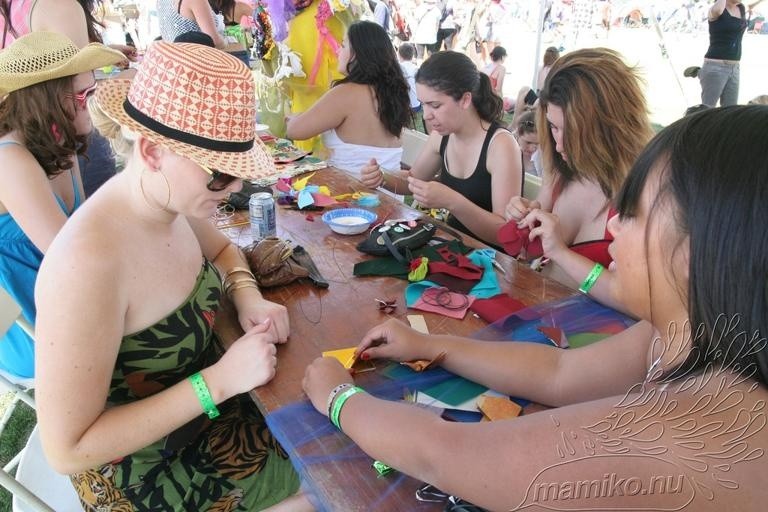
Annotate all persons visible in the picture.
[286,20,418,204]
[29,40,316,511]
[302,106,768,512]
[1,32,129,391]
[504,48,656,320]
[360,49,525,255]
[0,1,768,108]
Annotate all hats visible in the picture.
[96,41,276,180]
[0,28,127,96]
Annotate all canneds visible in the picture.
[248,191,276,242]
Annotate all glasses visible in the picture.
[199,166,237,192]
[64,82,98,107]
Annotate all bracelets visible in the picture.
[576,260,605,296]
[325,378,368,431]
[183,365,222,423]
[331,384,365,439]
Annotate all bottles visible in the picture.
[125,31,135,47]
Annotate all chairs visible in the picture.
[1,285,86,512]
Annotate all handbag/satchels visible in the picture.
[243,236,311,287]
[357,219,437,257]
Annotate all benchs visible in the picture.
[400,125,541,205]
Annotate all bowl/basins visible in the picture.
[322,208,378,235]
[255,123,269,137]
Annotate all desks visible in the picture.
[195,143,632,512]
